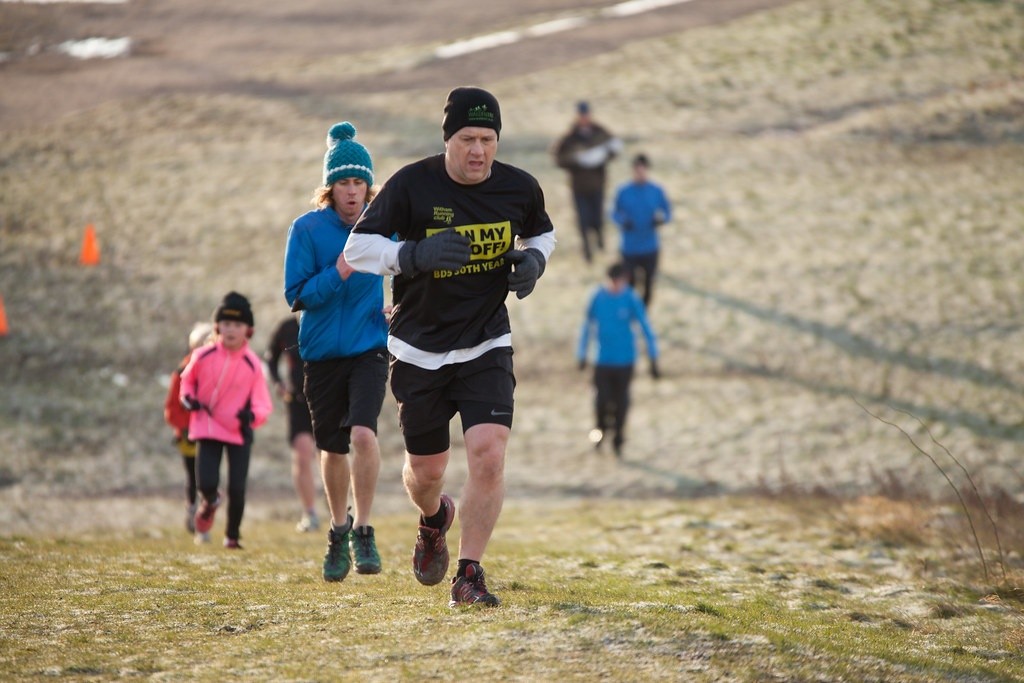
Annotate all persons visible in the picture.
[262,310,323,537]
[553,100,622,261]
[177,289,276,550]
[611,153,670,307]
[164,321,215,533]
[577,262,659,453]
[342,86,557,609]
[283,120,400,582]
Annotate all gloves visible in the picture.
[505,247,546,299]
[399,228,472,280]
[182,394,200,410]
[238,410,254,423]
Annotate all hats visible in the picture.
[321,122,373,188]
[216,292,254,326]
[442,87,502,141]
[189,323,214,350]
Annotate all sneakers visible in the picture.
[185,501,198,533]
[413,491,455,585]
[322,514,354,583]
[449,561,498,608]
[347,526,383,575]
[223,535,242,550]
[296,513,319,533]
[194,491,222,533]
[194,529,213,546]
